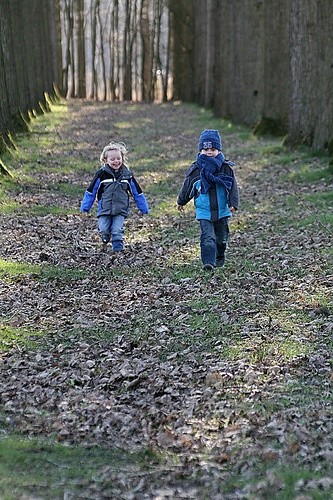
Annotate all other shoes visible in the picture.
[214,253,224,267]
[202,264,214,270]
[102,233,110,243]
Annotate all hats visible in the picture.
[198,129,222,152]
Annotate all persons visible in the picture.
[78,141,148,257]
[176,129,239,271]
